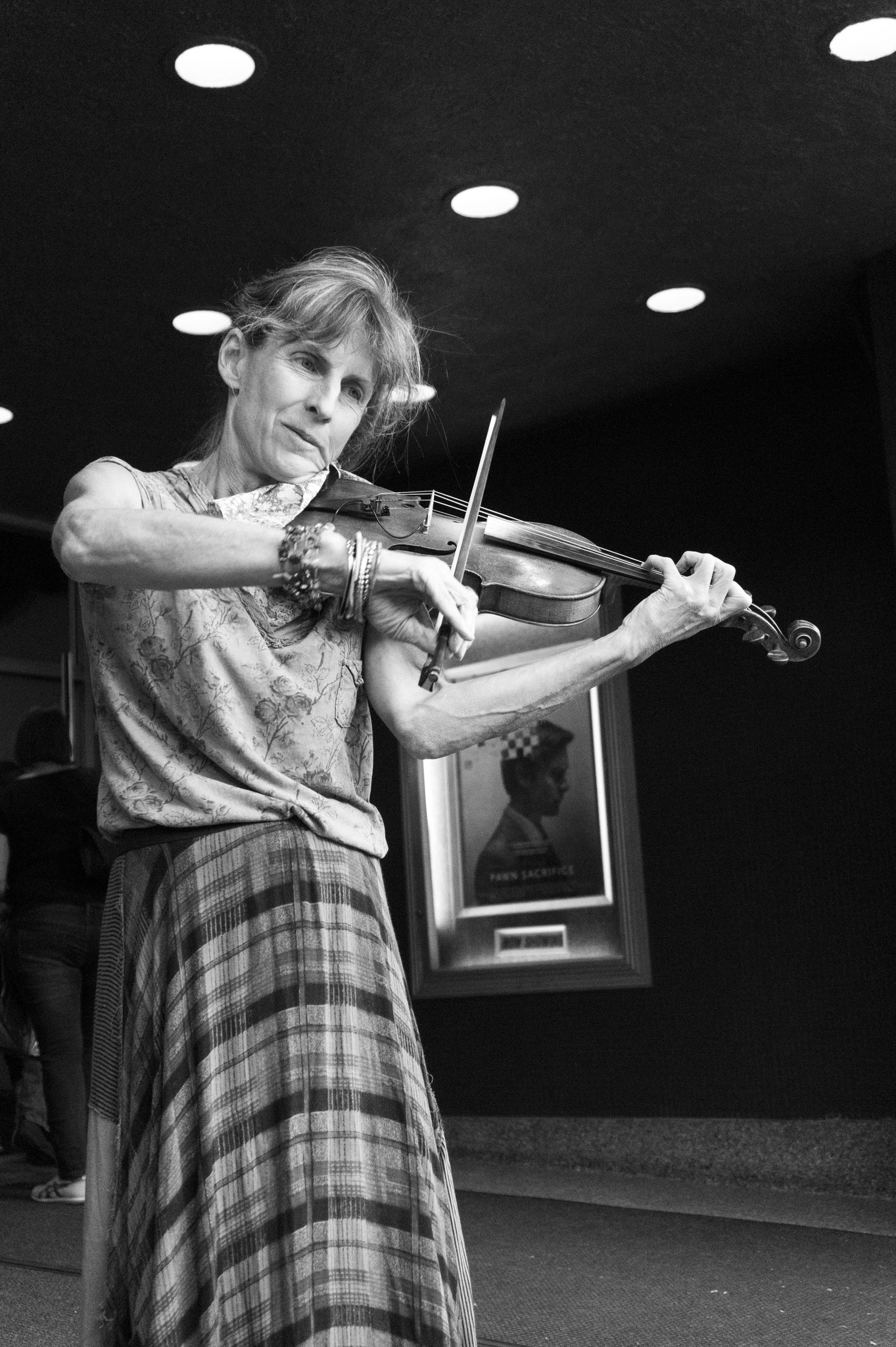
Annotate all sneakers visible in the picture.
[31,1174,87,1205]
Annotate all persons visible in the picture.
[0,713,95,1205]
[50,243,755,1347]
[474,720,580,906]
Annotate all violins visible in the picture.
[280,476,822,664]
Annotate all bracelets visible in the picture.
[282,522,382,625]
